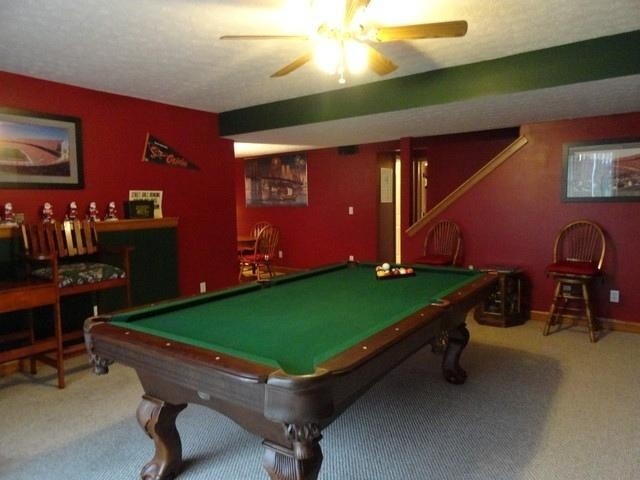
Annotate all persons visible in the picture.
[69,200,79,220]
[42,202,54,223]
[105,202,118,218]
[86,202,99,222]
[3,202,15,224]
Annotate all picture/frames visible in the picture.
[0,105,84,189]
[561,138,640,202]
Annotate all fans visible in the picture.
[219,1,467,77]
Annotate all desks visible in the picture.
[475,264,523,325]
[81,259,502,480]
[234,236,256,275]
[0,276,64,389]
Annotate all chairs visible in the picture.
[239,224,281,282]
[22,220,132,377]
[250,222,269,242]
[416,219,461,266]
[542,221,605,342]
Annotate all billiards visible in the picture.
[376,263,413,276]
